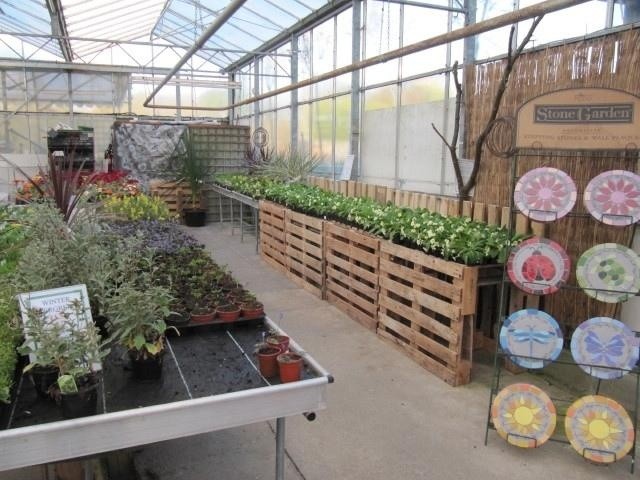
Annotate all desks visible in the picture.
[1,314,335,479]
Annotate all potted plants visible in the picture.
[103,291,175,379]
[56,301,121,418]
[209,170,304,209]
[256,334,305,383]
[3,124,269,337]
[18,308,71,398]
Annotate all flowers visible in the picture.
[307,184,533,267]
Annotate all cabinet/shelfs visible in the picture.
[484,87,640,476]
